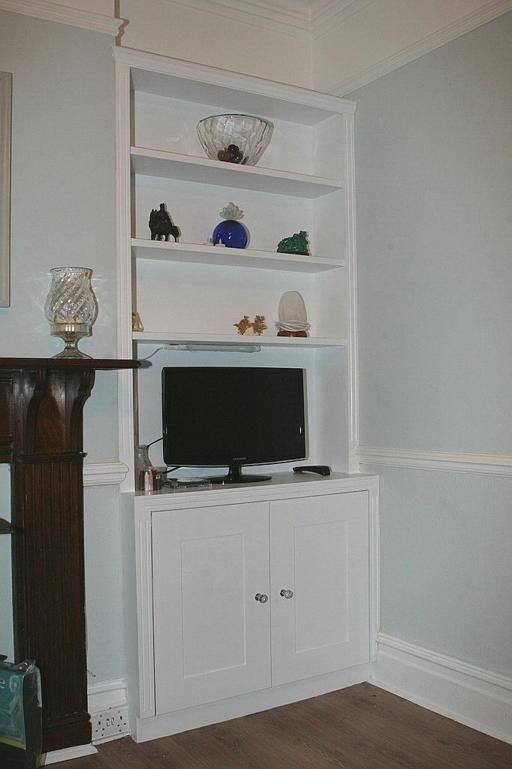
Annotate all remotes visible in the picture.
[293,466,330,476]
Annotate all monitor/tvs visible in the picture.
[161,365,309,484]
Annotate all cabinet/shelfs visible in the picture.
[112,42,364,492]
[127,471,379,743]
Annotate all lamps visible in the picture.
[45,265,97,360]
